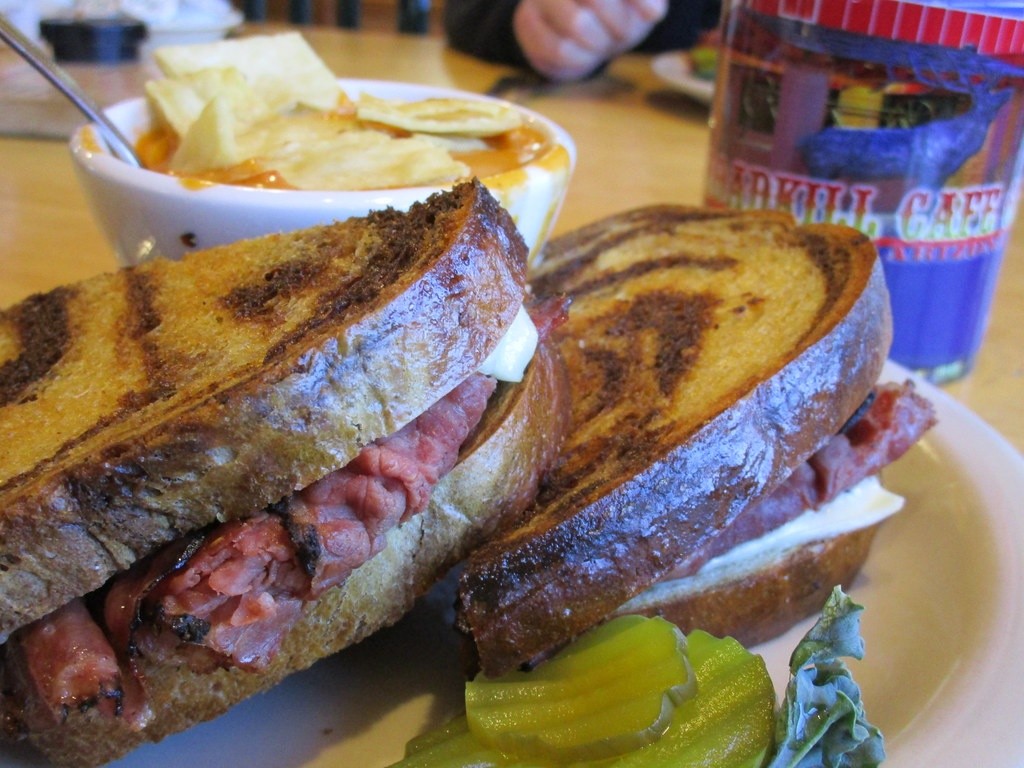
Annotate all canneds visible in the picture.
[696,0,1024,386]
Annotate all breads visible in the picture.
[0,181,896,768]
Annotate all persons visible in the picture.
[439,0,723,84]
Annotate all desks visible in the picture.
[0,27,1023,454]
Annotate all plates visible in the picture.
[652,53,717,100]
[77,356,1024,768]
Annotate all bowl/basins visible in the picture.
[71,80,573,275]
[10,10,246,48]
